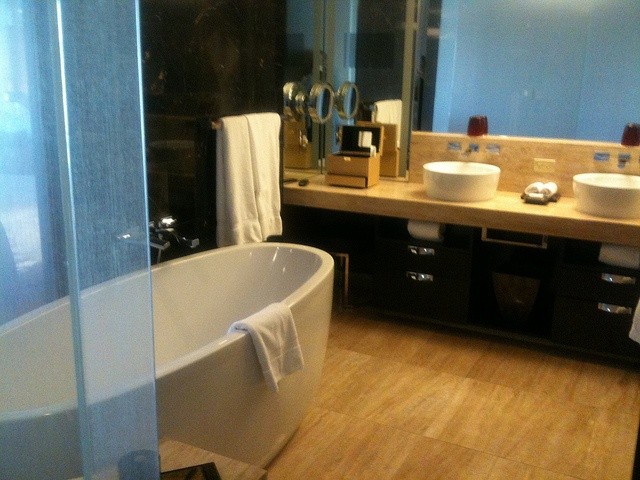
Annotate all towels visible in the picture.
[359,132,370,148]
[246,111,283,238]
[215,115,261,248]
[228,302,304,390]
[372,100,403,147]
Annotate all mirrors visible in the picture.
[320,0,417,179]
[337,123,383,156]
[410,1,640,144]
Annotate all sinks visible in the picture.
[572,173,640,218]
[423,160,501,200]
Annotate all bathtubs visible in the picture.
[0,244,334,480]
